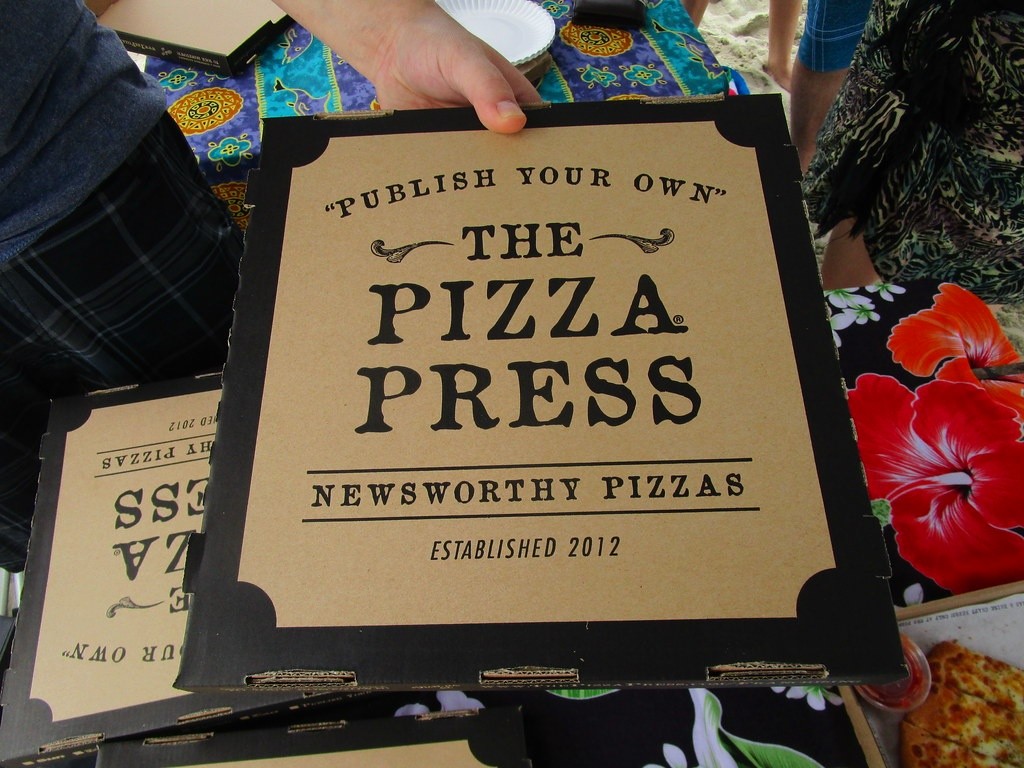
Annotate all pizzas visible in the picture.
[898,640,1024,768]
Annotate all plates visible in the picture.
[436,1,556,66]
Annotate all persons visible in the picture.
[684,0,1024,291]
[0,0,550,578]
